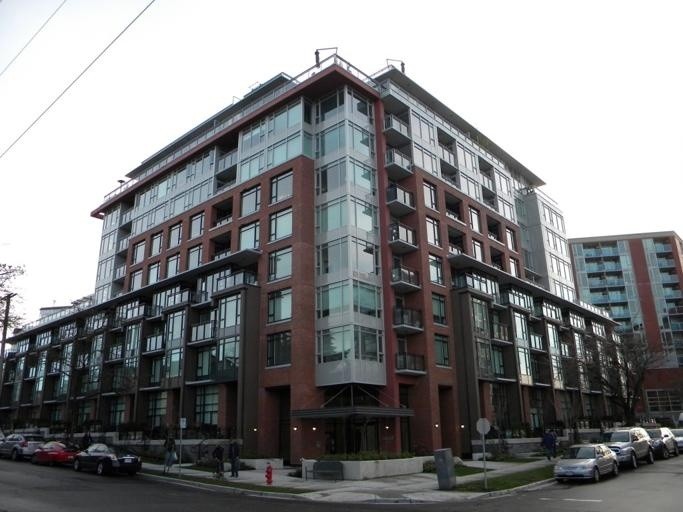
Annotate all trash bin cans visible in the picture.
[434,448,456,490]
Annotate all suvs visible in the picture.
[598,426,654,469]
[645,427,683,460]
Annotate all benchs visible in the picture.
[313,461,343,480]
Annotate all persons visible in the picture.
[227,441,240,479]
[212,442,225,479]
[540,428,555,460]
[161,432,178,476]
[79,433,90,450]
[550,429,560,455]
[323,429,336,454]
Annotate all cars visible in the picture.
[0,430,82,465]
[553,443,619,484]
[73,443,142,475]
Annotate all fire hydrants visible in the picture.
[265,465,273,486]
